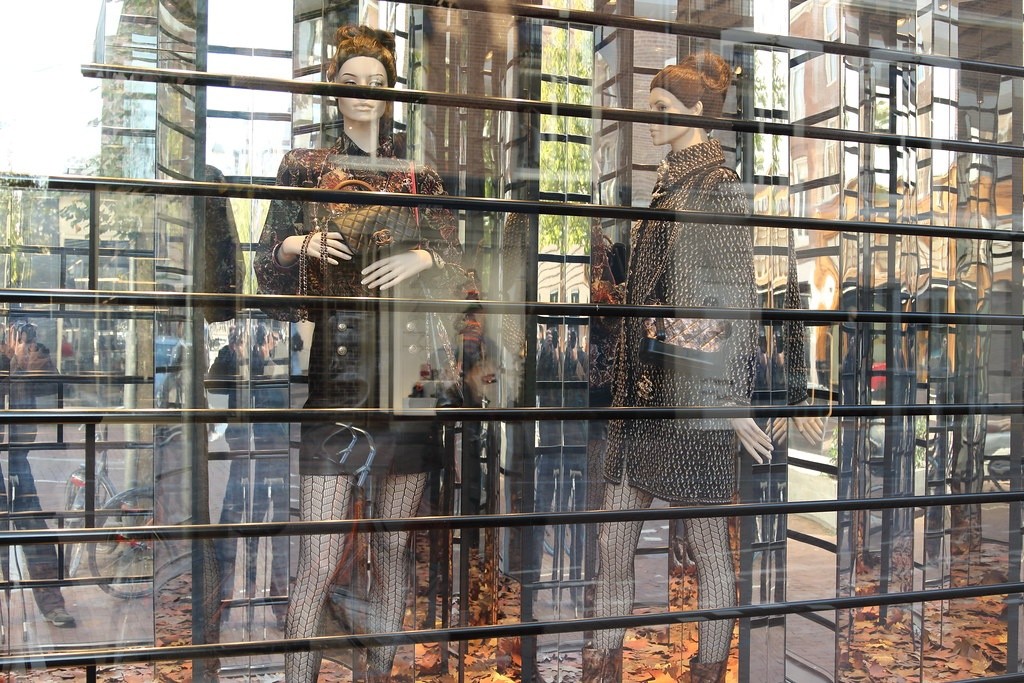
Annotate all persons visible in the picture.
[0,313,290,628]
[256,28,463,683]
[536,319,593,604]
[577,54,773,680]
[756,226,824,446]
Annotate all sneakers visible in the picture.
[42,607,75,626]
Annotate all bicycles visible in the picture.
[86,418,377,600]
[58,404,125,580]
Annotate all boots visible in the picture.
[689,657,728,683]
[582,641,623,683]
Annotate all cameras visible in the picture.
[232,324,265,345]
[11,322,35,343]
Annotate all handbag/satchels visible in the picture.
[328,180,424,258]
[638,297,732,376]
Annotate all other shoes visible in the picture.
[276,614,286,629]
